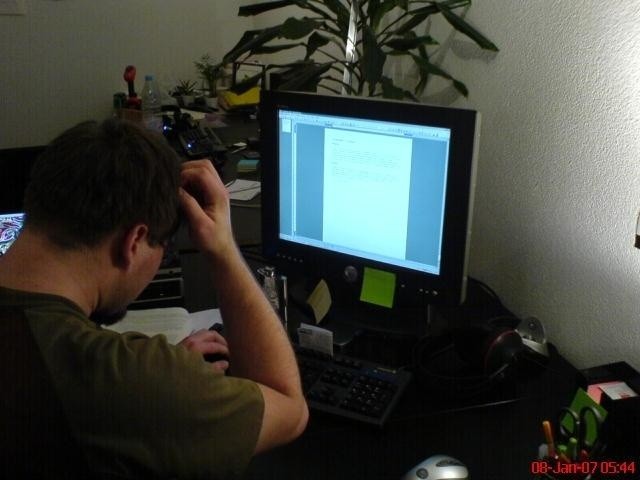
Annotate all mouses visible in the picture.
[400,454,471,480]
[178,118,198,129]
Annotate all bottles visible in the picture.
[141,75,163,136]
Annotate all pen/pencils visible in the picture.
[539,421,587,462]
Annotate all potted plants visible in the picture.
[170,77,198,107]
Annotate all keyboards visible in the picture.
[178,118,228,158]
[203,323,411,427]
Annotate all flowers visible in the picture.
[193,53,224,78]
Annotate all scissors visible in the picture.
[552,406,603,456]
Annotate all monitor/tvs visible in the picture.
[270,59,318,92]
[260,89,482,348]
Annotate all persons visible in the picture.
[0,119,312,479]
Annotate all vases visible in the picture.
[207,76,218,94]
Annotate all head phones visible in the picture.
[412,316,526,400]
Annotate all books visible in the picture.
[102,305,225,350]
